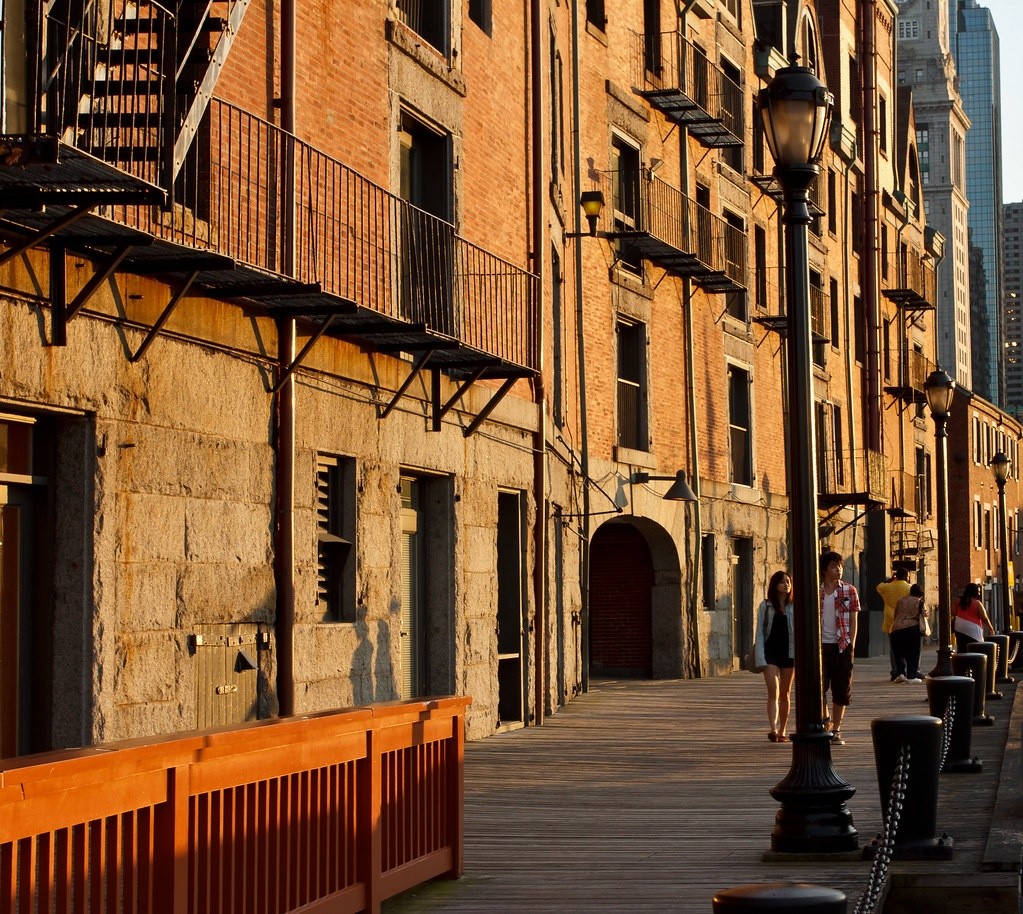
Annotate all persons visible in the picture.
[877,569,912,683]
[755,572,795,743]
[889,584,926,682]
[952,583,996,653]
[819,552,863,745]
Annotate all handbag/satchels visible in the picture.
[918,599,931,636]
[744,643,764,674]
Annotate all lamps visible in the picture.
[631,468,699,503]
[567,191,606,239]
[646,157,664,183]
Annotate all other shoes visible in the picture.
[778,736,787,742]
[822,716,831,731]
[917,673,925,679]
[768,732,777,742]
[829,736,845,744]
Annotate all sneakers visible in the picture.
[906,678,922,684]
[894,674,905,683]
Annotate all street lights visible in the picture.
[923,360,956,678]
[758,49,861,854]
[990,448,1013,633]
